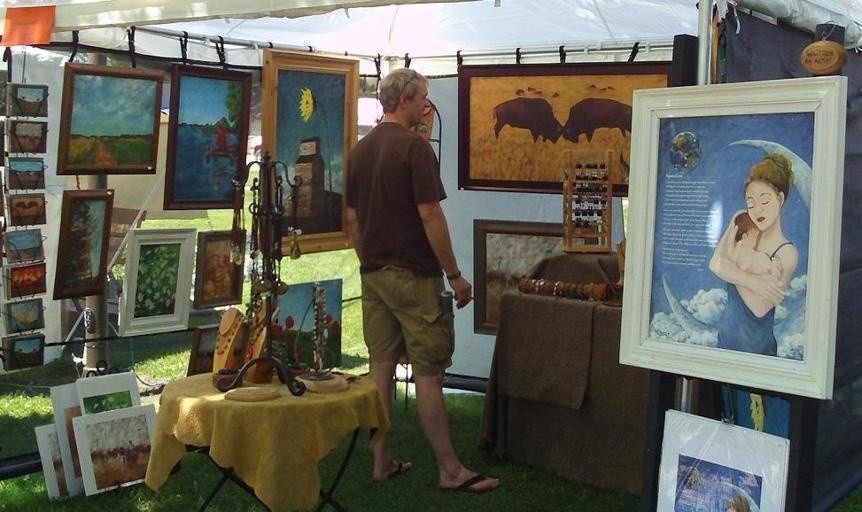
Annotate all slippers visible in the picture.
[440,473,499,493]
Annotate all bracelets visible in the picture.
[446,271,461,280]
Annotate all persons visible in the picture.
[341,70,505,489]
[708,151,799,355]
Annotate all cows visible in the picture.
[563,97,632,143]
[493,97,563,143]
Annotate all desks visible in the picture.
[144,369,392,512]
[477,290,650,498]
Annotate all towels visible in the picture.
[0,5,55,46]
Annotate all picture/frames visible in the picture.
[5,301,48,330]
[656,409,791,512]
[456,61,675,198]
[198,227,252,313]
[32,370,159,501]
[0,334,47,372]
[7,193,49,226]
[2,230,47,263]
[6,118,48,154]
[3,266,47,296]
[110,222,197,339]
[8,158,47,191]
[267,278,350,367]
[473,219,601,336]
[50,188,116,303]
[185,318,251,369]
[254,48,361,258]
[159,63,254,213]
[55,62,163,178]
[618,75,849,402]
[4,81,49,115]
